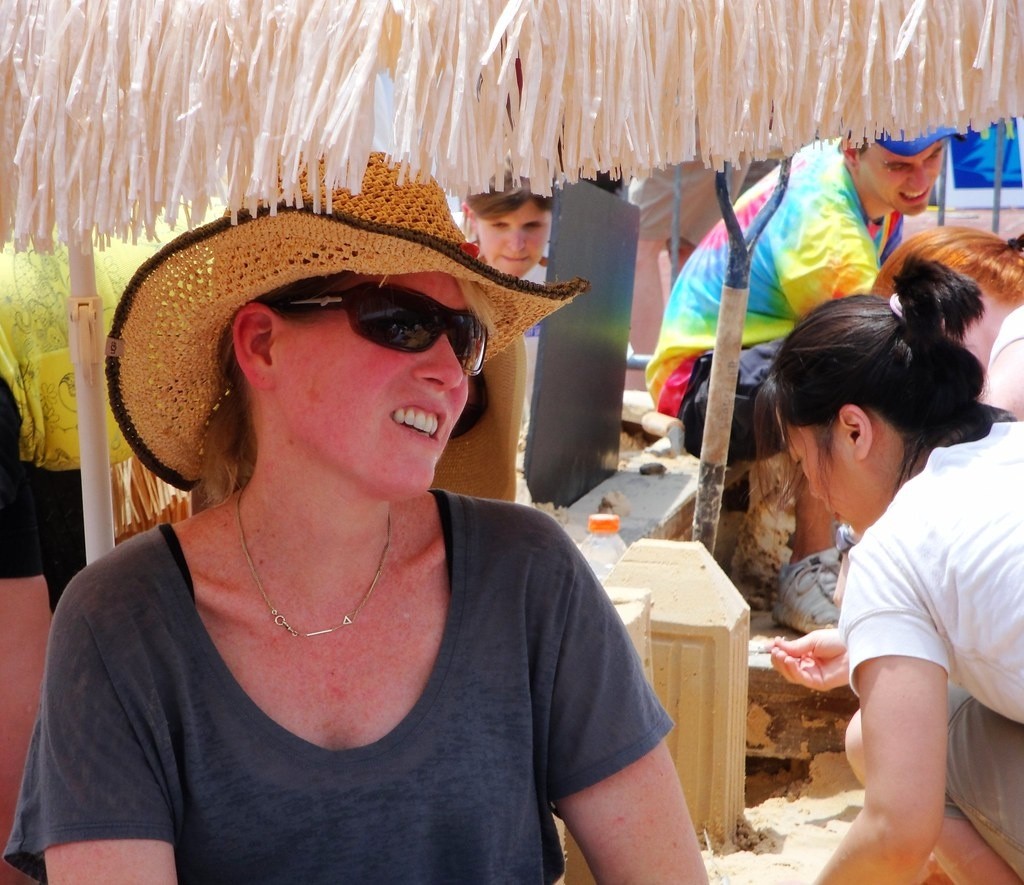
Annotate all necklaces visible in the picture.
[233,485,392,636]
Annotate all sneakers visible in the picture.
[772,546,844,634]
[836,524,856,553]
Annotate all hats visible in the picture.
[874,125,965,156]
[106,152,588,491]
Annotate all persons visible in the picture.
[0,375,55,883]
[462,172,552,338]
[643,125,1024,885]
[0,152,709,885]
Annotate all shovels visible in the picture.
[694,153,797,564]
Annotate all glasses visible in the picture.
[265,282,488,376]
